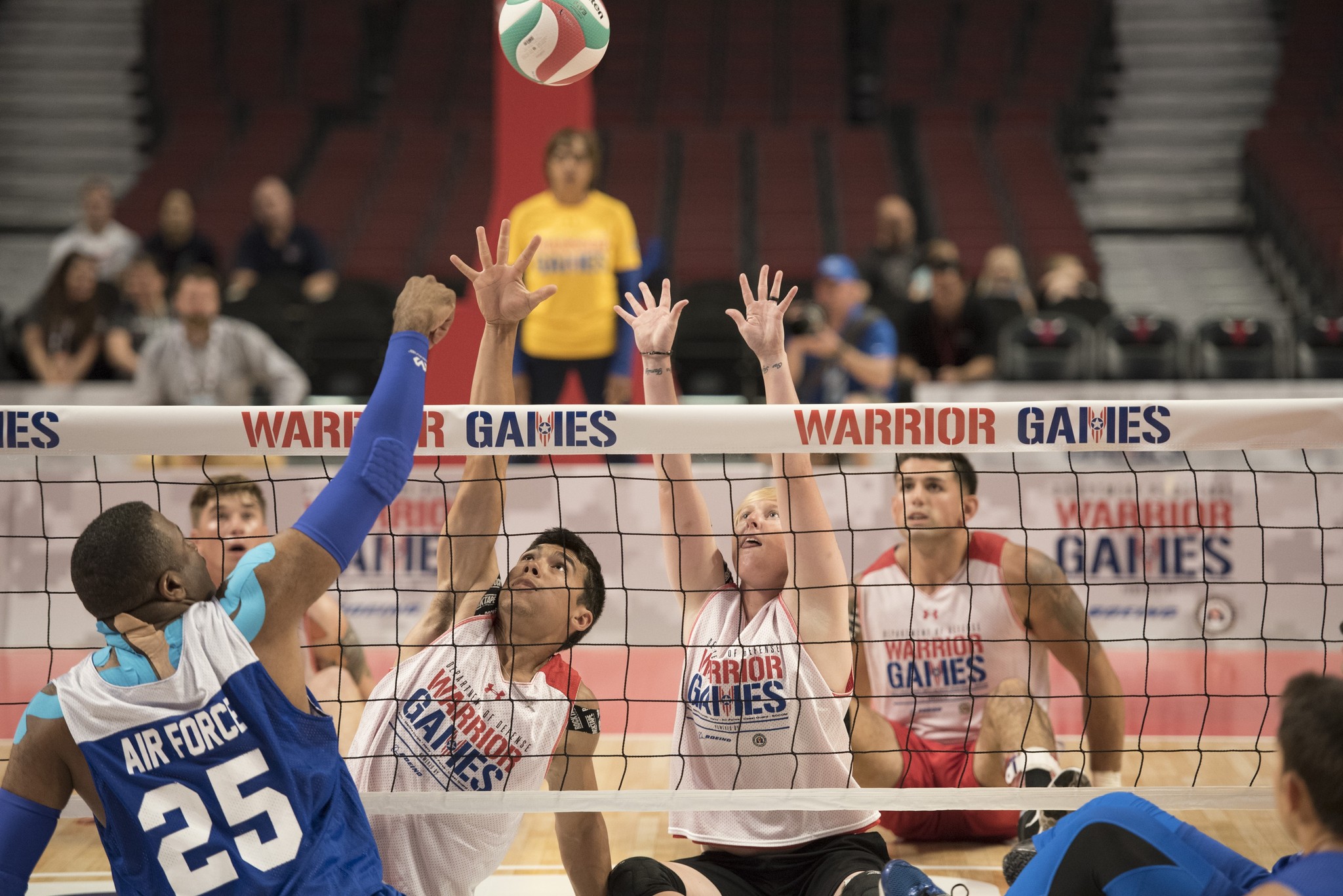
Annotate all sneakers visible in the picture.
[1003,839,1038,887]
[878,858,970,895]
[1017,768,1093,841]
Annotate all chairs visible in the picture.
[1242,0,1343,337]
[115,0,1102,314]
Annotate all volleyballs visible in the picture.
[498,0,610,87]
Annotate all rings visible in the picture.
[769,296,779,303]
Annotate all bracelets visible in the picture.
[640,349,674,357]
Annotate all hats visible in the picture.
[817,252,861,279]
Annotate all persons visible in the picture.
[2,175,339,407]
[784,193,1110,404]
[879,671,1343,896]
[605,265,889,896]
[844,453,1122,886]
[504,127,642,404]
[0,220,611,896]
